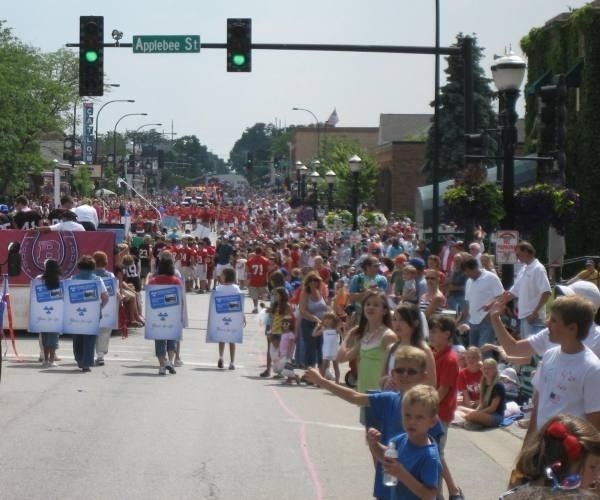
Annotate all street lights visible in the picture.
[71,81,123,169]
[131,123,164,187]
[113,113,149,169]
[292,107,321,158]
[292,159,337,222]
[348,153,362,230]
[93,99,136,164]
[150,131,179,170]
[489,42,529,305]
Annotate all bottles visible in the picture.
[383,443,398,484]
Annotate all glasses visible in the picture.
[393,367,421,375]
[425,276,436,280]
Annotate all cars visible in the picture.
[180,194,210,208]
[206,170,248,190]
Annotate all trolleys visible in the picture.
[344,353,357,387]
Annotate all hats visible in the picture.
[553,279,600,316]
[585,259,594,266]
[500,366,521,386]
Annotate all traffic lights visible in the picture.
[226,18,253,73]
[79,15,103,97]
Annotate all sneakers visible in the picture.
[217,358,224,368]
[157,357,185,376]
[38,352,61,368]
[127,282,281,330]
[76,356,105,371]
[228,363,235,370]
[259,368,343,387]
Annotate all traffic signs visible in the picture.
[132,35,201,55]
